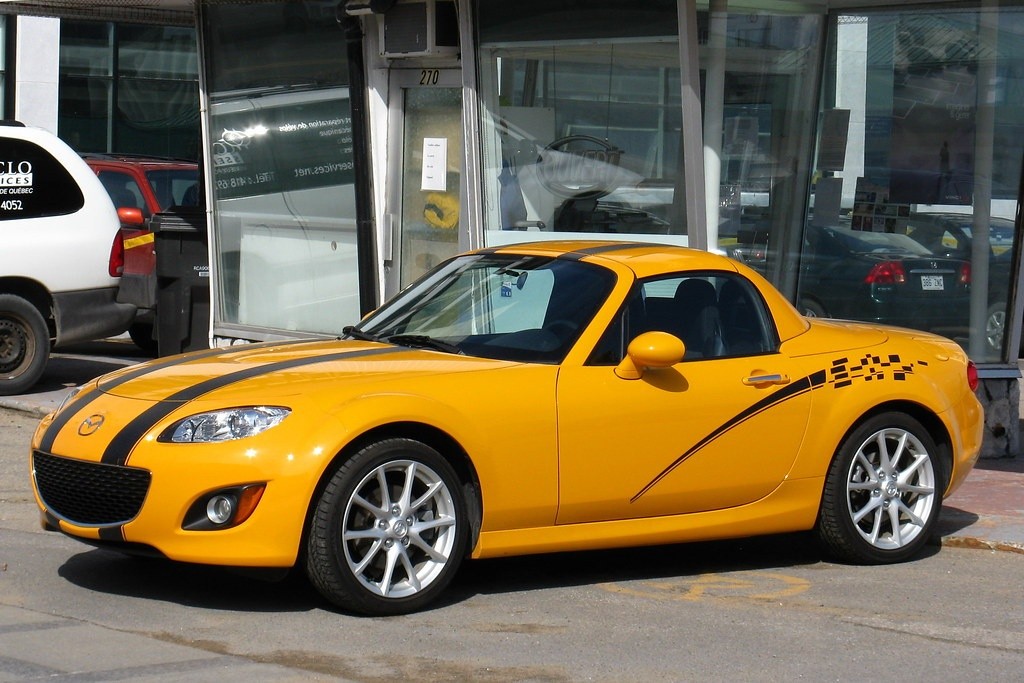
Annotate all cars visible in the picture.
[718,205,1024,357]
[28,240,986,616]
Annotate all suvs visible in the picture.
[208,83,645,333]
[1,118,210,395]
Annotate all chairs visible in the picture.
[666,279,725,361]
[554,273,634,365]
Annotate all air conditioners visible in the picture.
[378,0,459,59]
